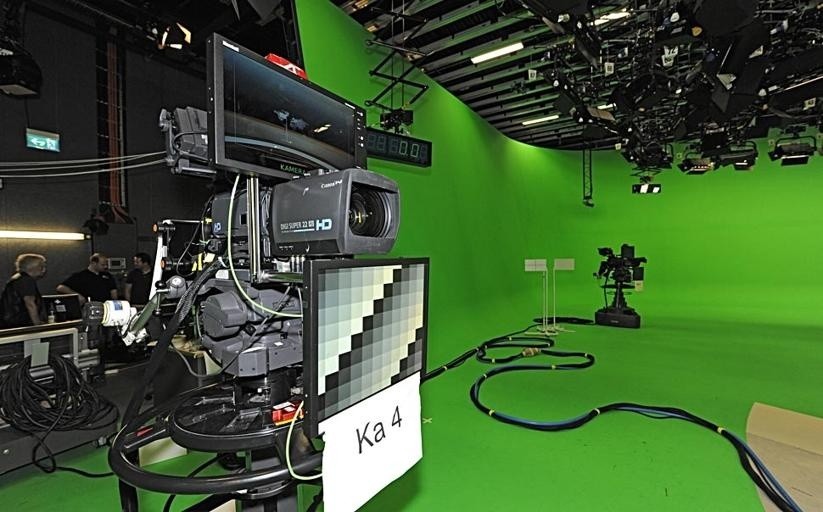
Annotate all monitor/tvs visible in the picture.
[24,127,62,154]
[206,33,367,187]
[42,294,82,322]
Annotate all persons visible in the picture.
[56,253,118,307]
[0,253,48,329]
[124,253,154,305]
